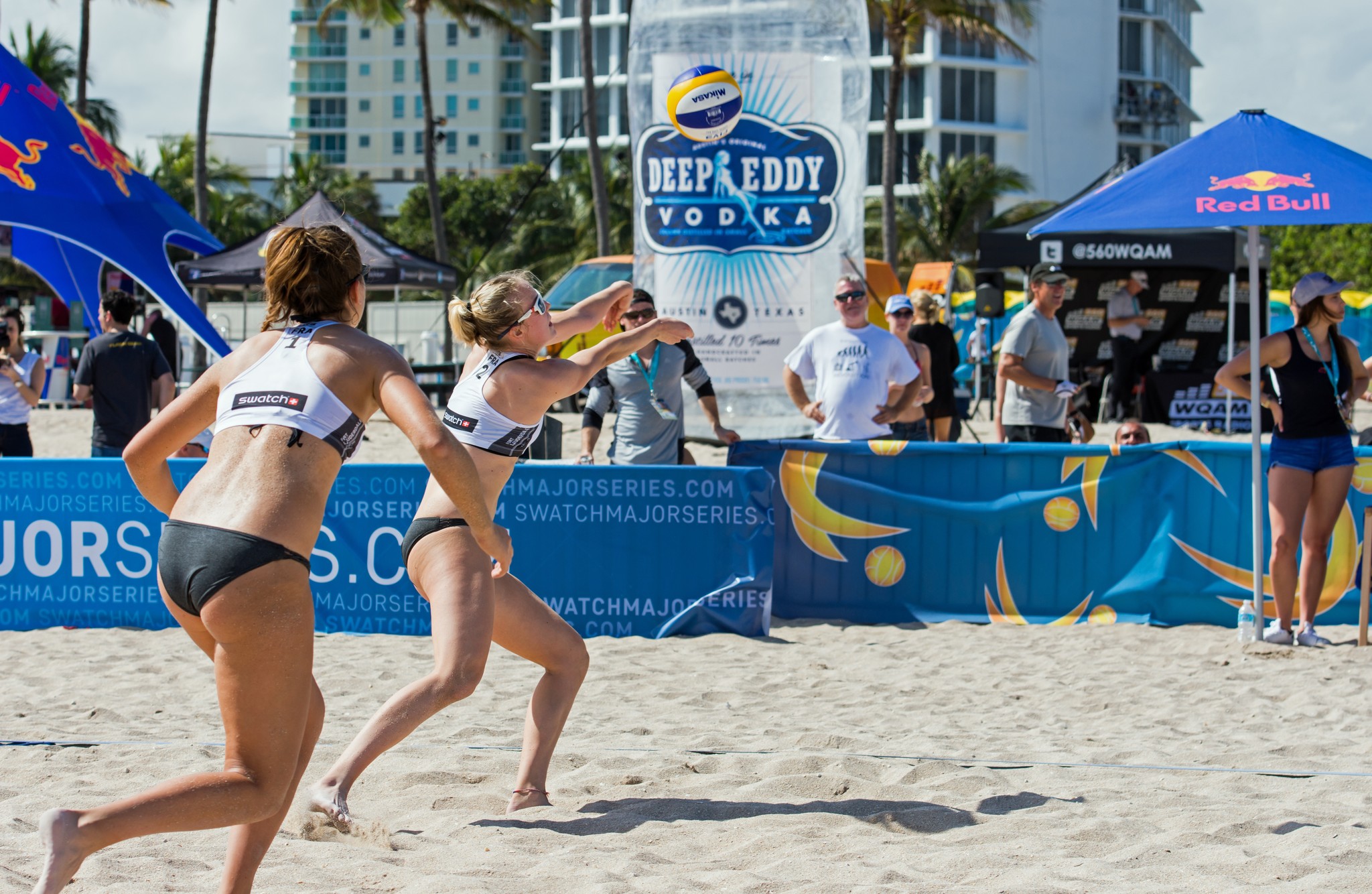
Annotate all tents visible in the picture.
[978,155,1273,435]
[0,43,232,359]
[171,189,454,385]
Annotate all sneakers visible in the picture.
[1295,621,1332,646]
[1251,619,1294,646]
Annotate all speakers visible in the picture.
[529,415,563,461]
[975,270,1005,318]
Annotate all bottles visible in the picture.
[1237,599,1256,646]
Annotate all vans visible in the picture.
[536,253,904,363]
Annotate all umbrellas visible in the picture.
[1028,107,1372,642]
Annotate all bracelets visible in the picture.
[1262,394,1273,409]
[1366,392,1372,401]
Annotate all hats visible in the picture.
[630,289,655,305]
[1029,262,1070,284]
[885,294,914,314]
[1293,273,1354,307]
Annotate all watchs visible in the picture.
[14,380,24,388]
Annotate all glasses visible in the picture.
[834,289,866,303]
[343,263,370,288]
[892,310,914,319]
[624,308,656,320]
[1048,278,1068,287]
[497,288,546,343]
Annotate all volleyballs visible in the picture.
[666,64,744,143]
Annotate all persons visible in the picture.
[302,272,692,816]
[1359,356,1372,402]
[883,293,935,442]
[908,284,962,444]
[1105,271,1157,421]
[578,289,741,468]
[149,307,182,399]
[26,224,514,894]
[0,305,46,456]
[72,290,175,456]
[1115,422,1152,445]
[964,260,1096,444]
[784,273,923,440]
[1214,270,1370,648]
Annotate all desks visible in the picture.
[411,362,464,382]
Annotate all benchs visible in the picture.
[418,383,456,407]
